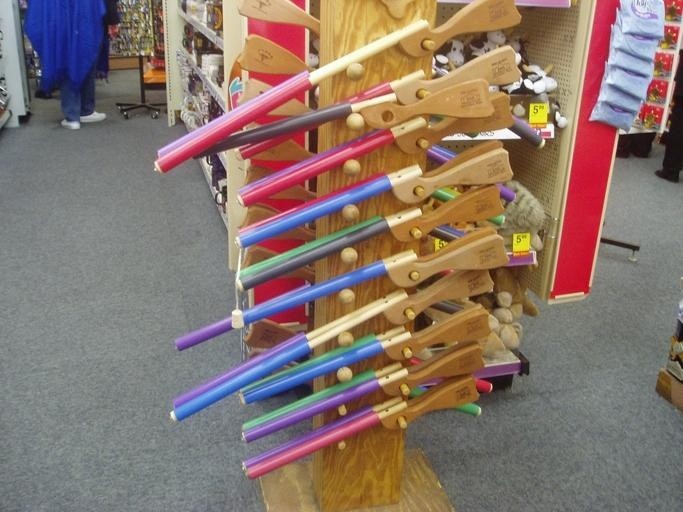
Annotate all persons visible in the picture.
[22,0,123,130]
[655,51,683,183]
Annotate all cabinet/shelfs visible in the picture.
[160,0,308,337]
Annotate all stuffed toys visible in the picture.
[431,25,569,129]
[473,268,541,359]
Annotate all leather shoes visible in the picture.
[655,171,680,182]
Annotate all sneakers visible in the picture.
[79,111,107,123]
[61,119,81,130]
